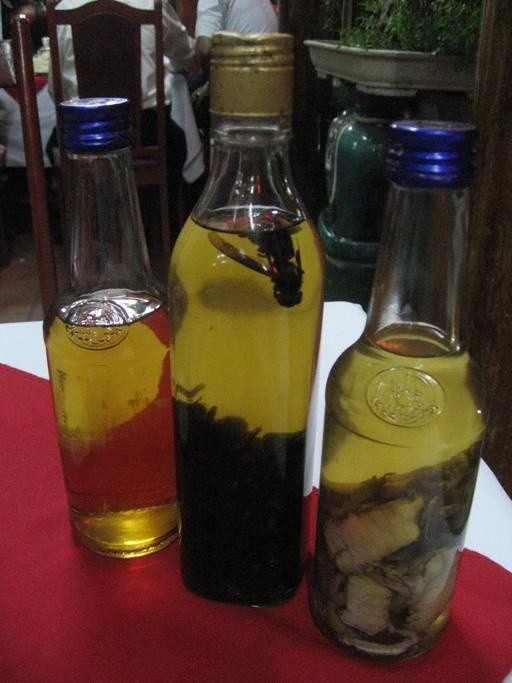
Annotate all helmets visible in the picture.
[30,66,51,77]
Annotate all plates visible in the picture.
[30,66,51,77]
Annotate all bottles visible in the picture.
[43,95,180,562]
[37,37,52,68]
[166,34,323,609]
[307,120,490,662]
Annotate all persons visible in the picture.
[49,2,199,247]
[187,1,276,128]
[2,2,49,267]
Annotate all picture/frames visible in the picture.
[2,298,512,682]
[1,35,200,166]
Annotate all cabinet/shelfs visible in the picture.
[37,37,52,68]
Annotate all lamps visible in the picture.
[19,0,186,312]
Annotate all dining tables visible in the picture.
[302,1,486,100]
